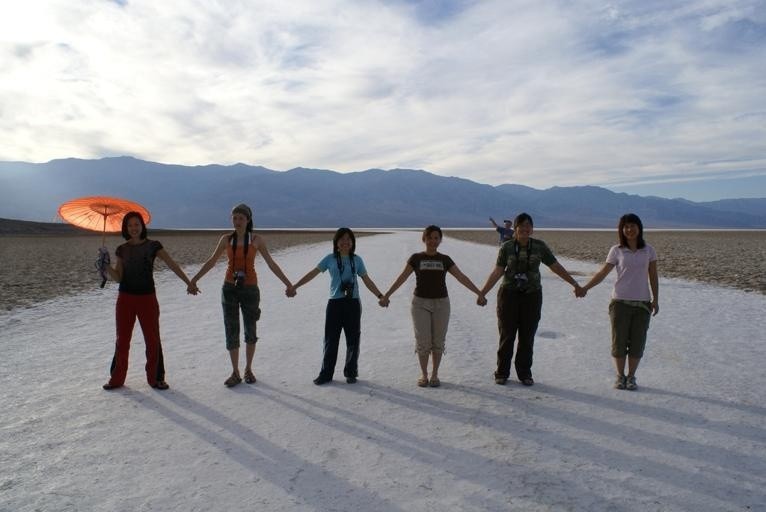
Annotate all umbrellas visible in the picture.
[58,196,151,250]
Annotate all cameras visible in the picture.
[233,272,247,287]
[340,283,354,298]
[514,274,528,288]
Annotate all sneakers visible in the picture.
[313,374,334,385]
[345,375,358,384]
[103,378,123,390]
[519,373,534,387]
[147,379,169,391]
[494,373,509,385]
[613,374,638,391]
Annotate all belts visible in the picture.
[615,298,653,313]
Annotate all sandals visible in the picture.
[223,369,240,387]
[243,366,258,385]
[417,375,441,388]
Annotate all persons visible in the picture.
[186,203,296,387]
[574,213,659,389]
[489,217,515,247]
[286,228,390,385]
[379,224,487,387]
[97,210,201,390]
[477,212,587,386]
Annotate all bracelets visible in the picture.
[195,276,199,281]
[378,293,383,298]
